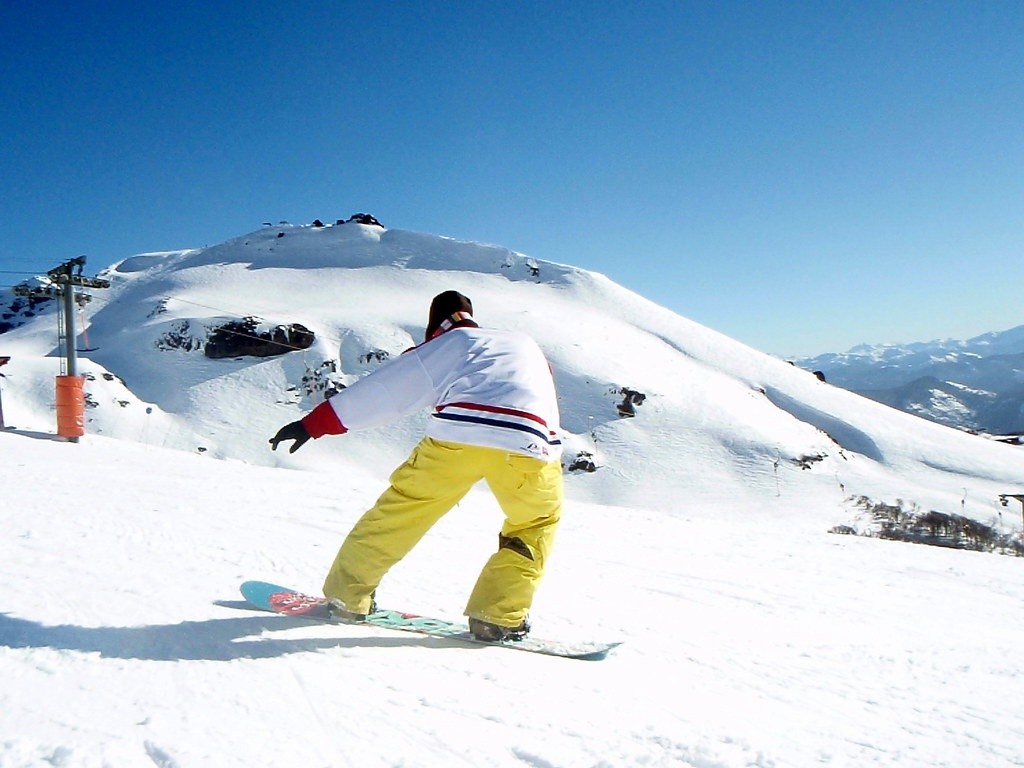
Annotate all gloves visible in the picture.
[269,420,311,454]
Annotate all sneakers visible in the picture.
[468,616,531,642]
[327,601,377,622]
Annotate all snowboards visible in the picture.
[239,580,627,662]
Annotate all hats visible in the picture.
[424,290,478,341]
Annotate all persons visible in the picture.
[268,290,563,643]
